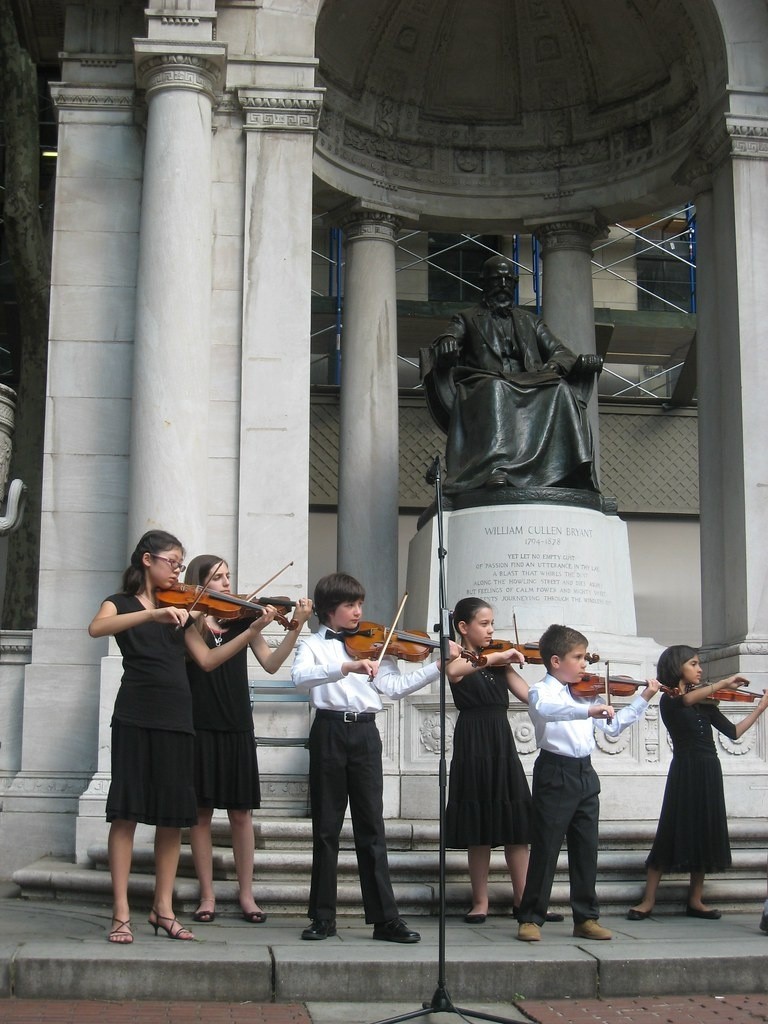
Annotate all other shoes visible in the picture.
[627,909,652,920]
[464,906,489,923]
[512,904,564,922]
[686,903,721,919]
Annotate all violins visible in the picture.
[692,680,763,702]
[154,583,320,632]
[481,637,600,666]
[343,618,490,668]
[569,670,681,699]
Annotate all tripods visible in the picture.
[372,461,526,1024]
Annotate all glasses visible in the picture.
[150,552,186,572]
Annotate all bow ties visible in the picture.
[325,629,344,643]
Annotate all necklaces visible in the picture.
[201,613,223,647]
[138,593,162,609]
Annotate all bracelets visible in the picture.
[710,683,715,700]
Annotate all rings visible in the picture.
[300,603,306,607]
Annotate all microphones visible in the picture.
[426,456,440,485]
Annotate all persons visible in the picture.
[291,573,463,944]
[517,623,663,942]
[185,555,312,924]
[628,645,768,932]
[428,255,604,495]
[88,529,278,944]
[446,597,564,924]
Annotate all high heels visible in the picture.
[108,915,134,944]
[148,908,192,939]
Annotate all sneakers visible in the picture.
[573,919,612,940]
[517,923,541,941]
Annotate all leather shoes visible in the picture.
[302,918,337,940]
[373,917,421,942]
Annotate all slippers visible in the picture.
[192,903,216,922]
[241,908,267,923]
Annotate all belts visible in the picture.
[316,708,375,722]
